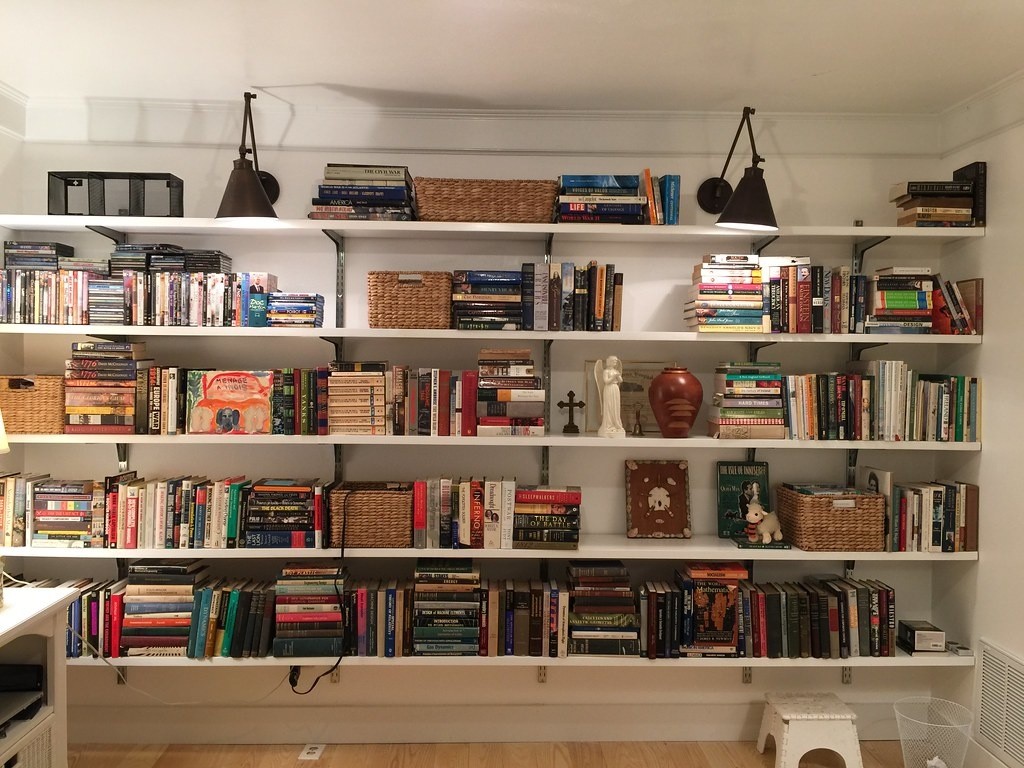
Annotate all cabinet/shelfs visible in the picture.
[0,586,80,768]
[0,212,983,768]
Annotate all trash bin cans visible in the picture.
[894,696,974,768]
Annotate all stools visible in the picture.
[756,691,864,768]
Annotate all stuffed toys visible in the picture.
[744,504,783,544]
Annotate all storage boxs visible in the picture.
[897,619,945,652]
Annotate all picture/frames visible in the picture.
[623,459,692,539]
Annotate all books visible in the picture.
[0,472,581,550]
[708,359,981,442]
[729,537,792,549]
[888,162,987,227]
[783,465,980,552]
[554,168,681,225]
[62,342,545,436]
[3,558,896,660]
[682,254,984,336]
[452,260,623,332]
[0,241,325,328]
[308,163,415,221]
[626,459,692,539]
[716,461,769,539]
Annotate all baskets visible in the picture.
[367,271,453,329]
[329,480,414,548]
[413,177,559,223]
[776,485,886,552]
[0,374,65,434]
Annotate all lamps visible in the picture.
[213,92,280,219]
[697,107,780,232]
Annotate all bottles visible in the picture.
[647,366,703,438]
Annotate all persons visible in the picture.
[250,276,264,294]
[868,471,879,493]
[237,280,241,289]
[601,356,624,432]
